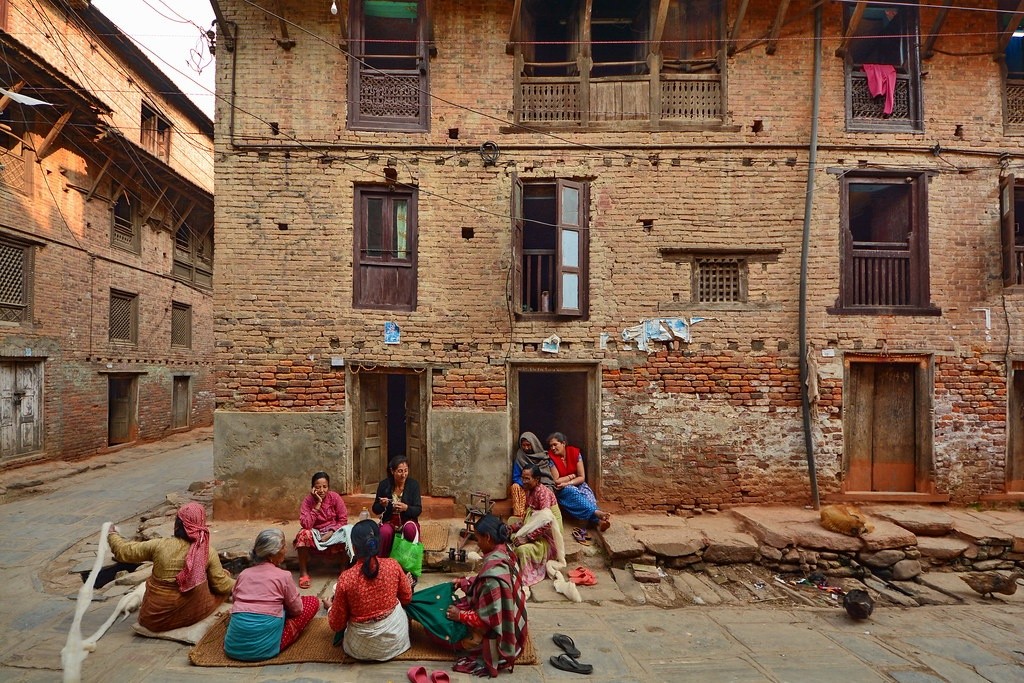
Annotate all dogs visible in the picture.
[820,504,875,538]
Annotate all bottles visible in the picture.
[358,506,371,521]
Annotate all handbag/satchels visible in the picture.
[388,521,423,577]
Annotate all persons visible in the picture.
[322,520,413,661]
[292,472,347,590]
[423,514,528,677]
[223,528,320,662]
[546,432,611,532]
[108,502,236,633]
[507,463,563,587]
[511,432,559,518]
[372,455,422,586]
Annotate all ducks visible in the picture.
[958,571,1024,600]
[843,589,873,621]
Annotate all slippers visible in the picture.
[550,654,593,673]
[407,666,432,683]
[574,527,592,539]
[569,573,597,585]
[299,575,311,588]
[432,669,450,683]
[553,633,581,658]
[573,531,590,545]
[568,566,596,578]
